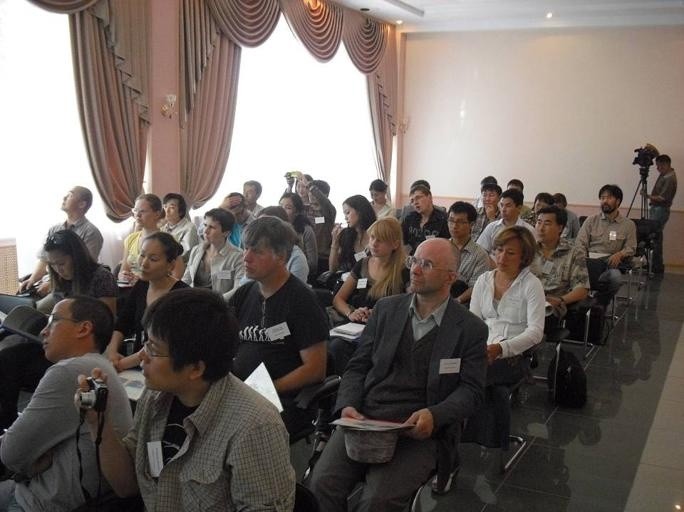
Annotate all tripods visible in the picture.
[626,179,648,220]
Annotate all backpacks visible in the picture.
[547,349,589,411]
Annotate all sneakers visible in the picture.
[433,466,458,493]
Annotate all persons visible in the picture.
[0,169,341,437]
[74,287,297,512]
[638,154,678,277]
[300,238,489,511]
[0,295,134,510]
[323,175,589,438]
[570,183,640,349]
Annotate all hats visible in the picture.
[330,416,415,462]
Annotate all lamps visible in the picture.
[160,92,178,120]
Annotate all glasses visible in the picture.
[46,314,82,323]
[140,331,169,358]
[405,255,453,274]
[260,302,269,329]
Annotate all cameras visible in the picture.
[287,172,300,178]
[78,376,107,412]
